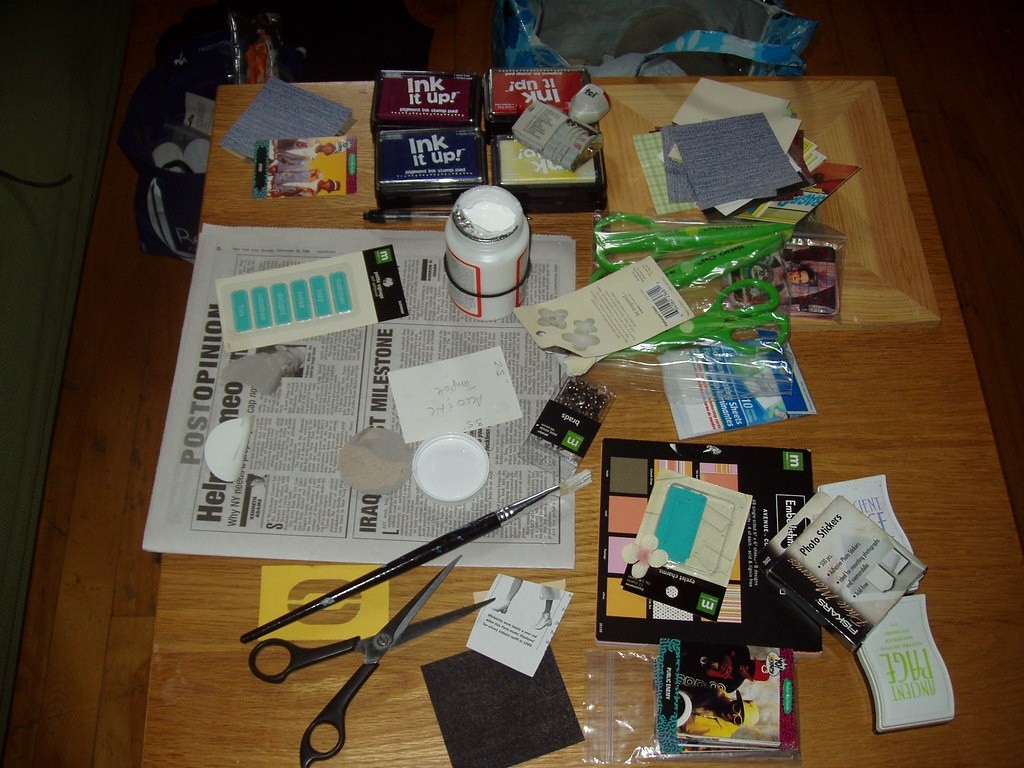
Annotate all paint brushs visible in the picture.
[240,468,593,645]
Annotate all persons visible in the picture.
[268,138,341,197]
[493,578,562,630]
[255,344,306,377]
[730,254,817,304]
[678,639,770,738]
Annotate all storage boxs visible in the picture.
[372,69,608,212]
[764,494,927,652]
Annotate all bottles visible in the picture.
[445,185,530,321]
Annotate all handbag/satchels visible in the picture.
[492,0,817,80]
[116,0,435,264]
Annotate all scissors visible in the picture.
[617,278,791,357]
[590,213,794,291]
[249,554,496,768]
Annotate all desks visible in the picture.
[141,77,1024,768]
